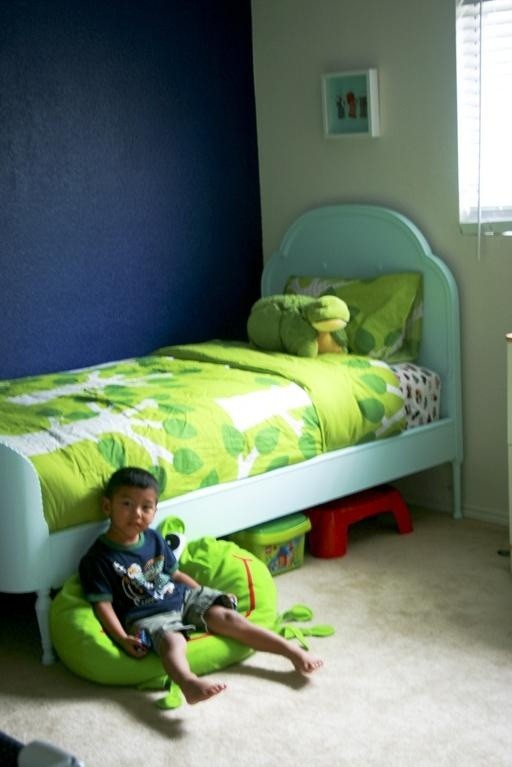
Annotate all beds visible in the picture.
[0,204,466,666]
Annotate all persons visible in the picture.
[78,466,323,705]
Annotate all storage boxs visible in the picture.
[226,513,312,577]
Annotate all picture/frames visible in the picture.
[319,68,380,140]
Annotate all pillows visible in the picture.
[282,271,425,361]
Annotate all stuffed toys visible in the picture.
[245,293,351,358]
